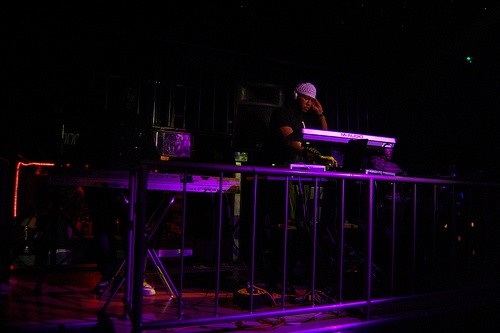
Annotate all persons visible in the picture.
[271,82,338,221]
[108,83,150,169]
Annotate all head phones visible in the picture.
[294,90,298,100]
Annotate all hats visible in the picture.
[294,82,317,101]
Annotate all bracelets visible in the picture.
[317,113,324,119]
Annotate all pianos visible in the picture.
[68,166,241,195]
